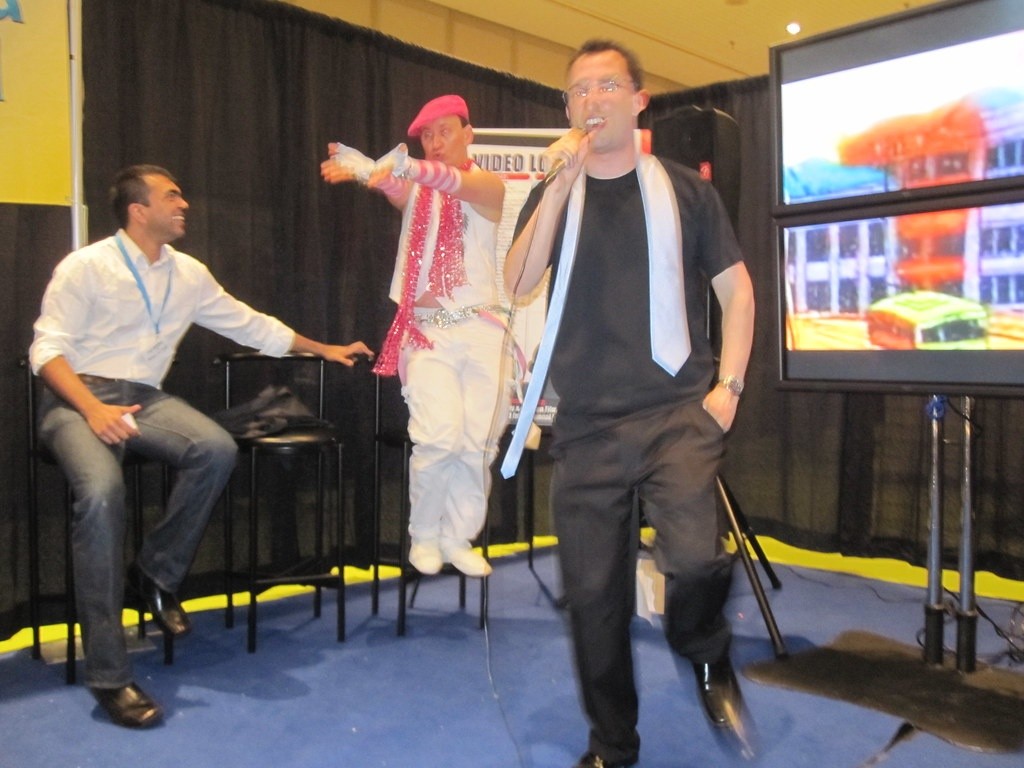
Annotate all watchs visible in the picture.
[717,373,743,398]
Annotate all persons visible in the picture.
[320,91,518,578]
[502,35,755,767]
[28,165,375,731]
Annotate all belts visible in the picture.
[32,373,119,387]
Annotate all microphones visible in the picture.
[544,127,588,186]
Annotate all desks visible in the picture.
[502,423,552,569]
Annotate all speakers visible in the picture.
[651,105,741,244]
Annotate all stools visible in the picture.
[21,354,177,684]
[212,351,347,654]
[371,370,490,638]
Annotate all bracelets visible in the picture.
[397,167,411,179]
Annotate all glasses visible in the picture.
[561,80,632,105]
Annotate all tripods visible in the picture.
[709,473,790,664]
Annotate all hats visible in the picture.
[408,94,469,137]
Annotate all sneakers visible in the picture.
[446,547,493,577]
[408,538,444,576]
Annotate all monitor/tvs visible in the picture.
[769,0,1024,217]
[770,190,1024,397]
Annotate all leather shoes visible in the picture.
[691,652,741,729]
[90,682,163,729]
[575,749,638,768]
[125,564,193,638]
